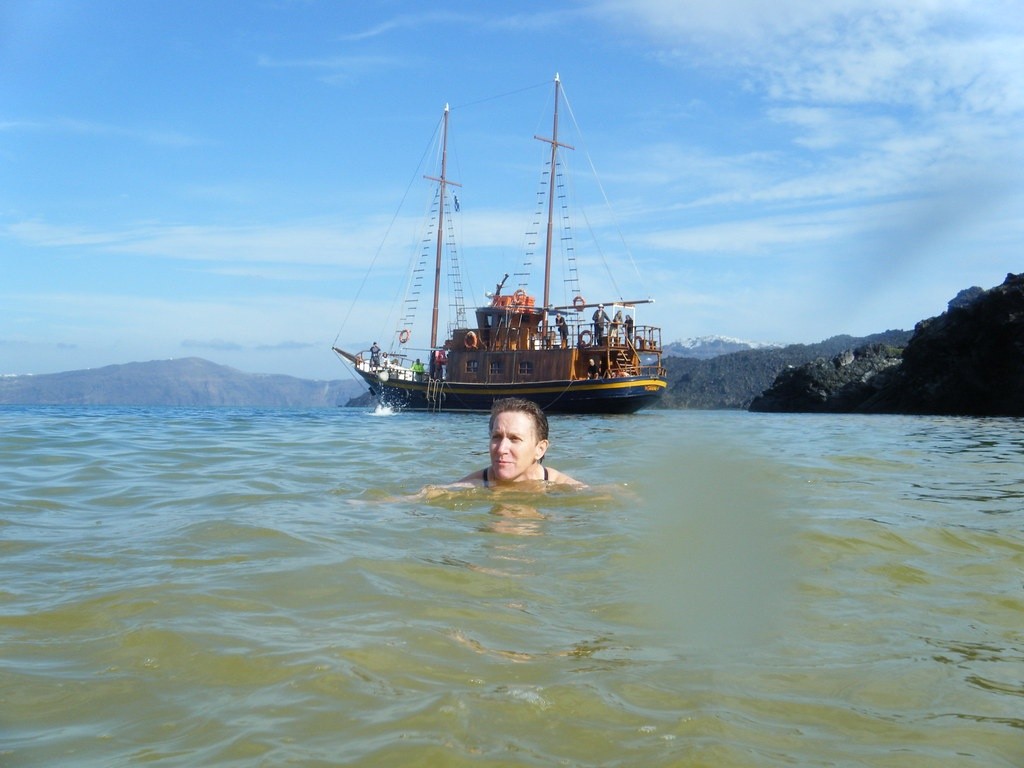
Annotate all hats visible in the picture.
[598,304,604,308]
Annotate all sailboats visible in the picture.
[332,73,667,415]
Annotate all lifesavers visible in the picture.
[464,331,478,350]
[578,329,593,348]
[399,328,410,343]
[573,295,586,311]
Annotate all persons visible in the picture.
[592,304,612,346]
[556,313,569,346]
[609,311,623,339]
[370,342,381,367]
[455,399,581,487]
[411,359,425,383]
[587,359,629,380]
[624,315,633,345]
[430,353,445,381]
